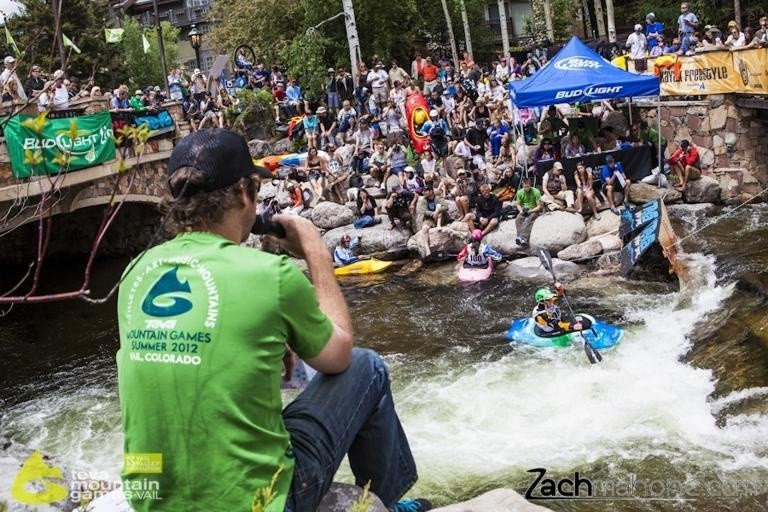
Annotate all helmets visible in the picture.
[471,229,483,241]
[534,288,557,302]
[341,234,351,243]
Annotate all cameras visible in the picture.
[198,73,202,76]
[271,201,278,205]
[458,180,463,183]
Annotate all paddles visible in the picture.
[538,247,602,365]
[433,250,519,259]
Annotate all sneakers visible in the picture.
[391,499,430,511]
[516,237,528,247]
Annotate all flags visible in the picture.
[142,32,150,54]
[4,25,22,59]
[104,27,126,43]
[61,32,80,53]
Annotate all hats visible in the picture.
[167,128,271,200]
[3,54,297,99]
[306,13,738,214]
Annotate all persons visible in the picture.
[111,124,434,512]
[512,177,542,247]
[415,184,450,258]
[234,49,666,236]
[0,54,232,146]
[622,0,767,74]
[333,235,370,268]
[456,229,510,267]
[667,139,702,192]
[529,282,590,335]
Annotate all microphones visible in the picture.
[250,215,285,235]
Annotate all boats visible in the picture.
[456,256,492,284]
[251,150,332,177]
[404,90,434,153]
[331,255,394,275]
[504,313,624,353]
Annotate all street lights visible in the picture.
[186,22,202,69]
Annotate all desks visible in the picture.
[536,141,654,196]
